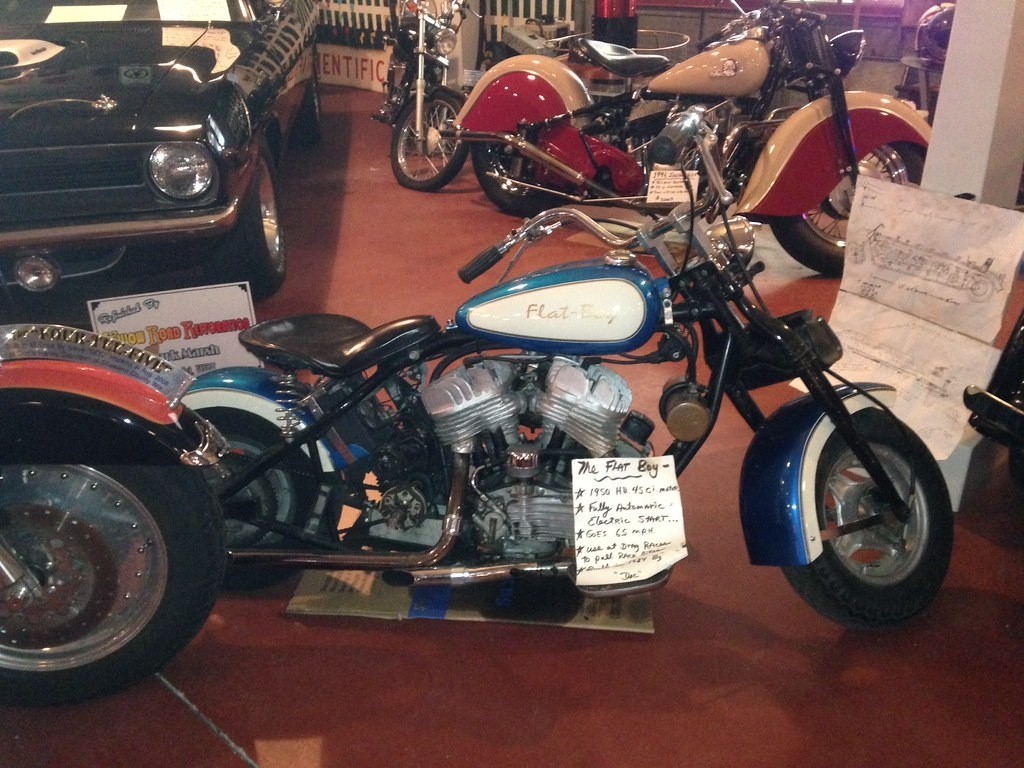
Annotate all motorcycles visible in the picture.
[0,98,955,709]
[452,0,934,278]
[372,0,474,192]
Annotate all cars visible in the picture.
[1,0,329,313]
